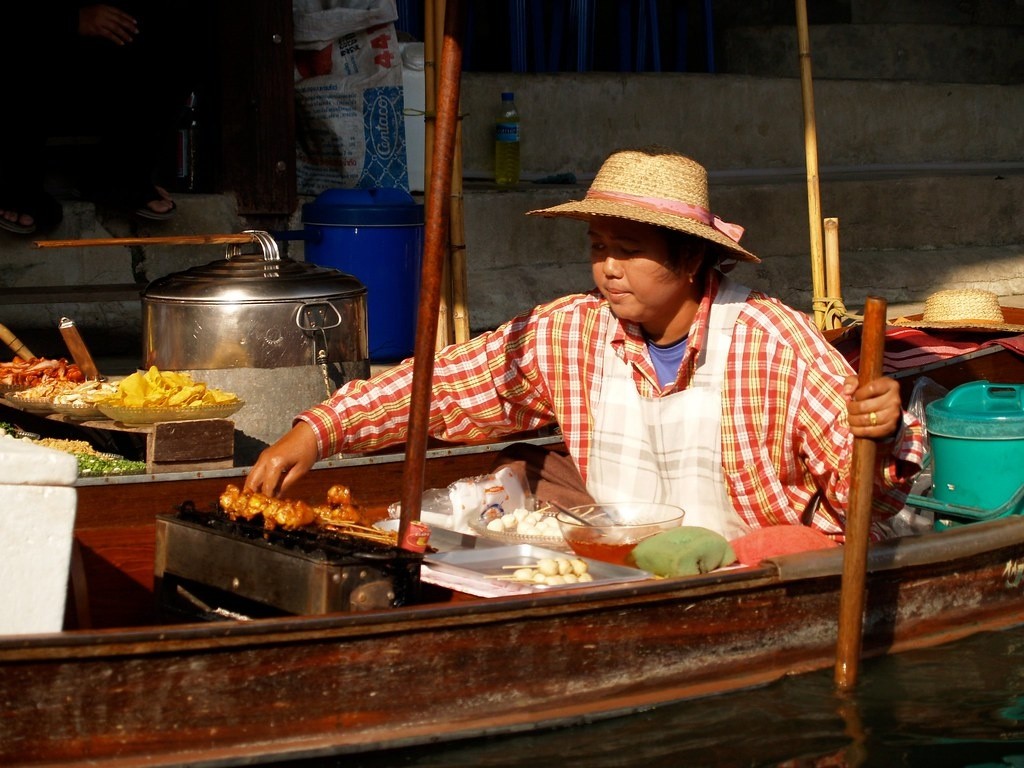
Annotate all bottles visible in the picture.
[493,91,524,184]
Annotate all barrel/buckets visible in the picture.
[299,184,424,365]
[900,379,1024,532]
[398,41,426,193]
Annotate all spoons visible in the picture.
[547,497,606,535]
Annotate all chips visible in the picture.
[103,366,243,407]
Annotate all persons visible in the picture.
[239,149,927,550]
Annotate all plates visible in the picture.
[422,543,654,593]
[3,390,56,414]
[96,399,248,426]
[0,385,31,400]
[467,516,571,550]
[48,400,111,422]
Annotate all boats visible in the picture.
[1,320,1024,765]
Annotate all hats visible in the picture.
[885,287,1023,337]
[524,148,764,263]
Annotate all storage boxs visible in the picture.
[141,229,370,468]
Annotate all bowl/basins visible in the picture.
[552,501,685,569]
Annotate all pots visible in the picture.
[140,226,372,367]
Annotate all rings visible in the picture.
[869,412,875,427]
